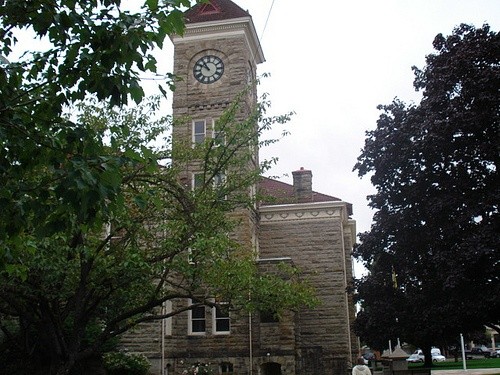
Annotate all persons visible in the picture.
[352,358,372,375]
[193,363,200,375]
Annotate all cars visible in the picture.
[405,340,500,364]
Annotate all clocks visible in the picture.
[192,55,224,84]
